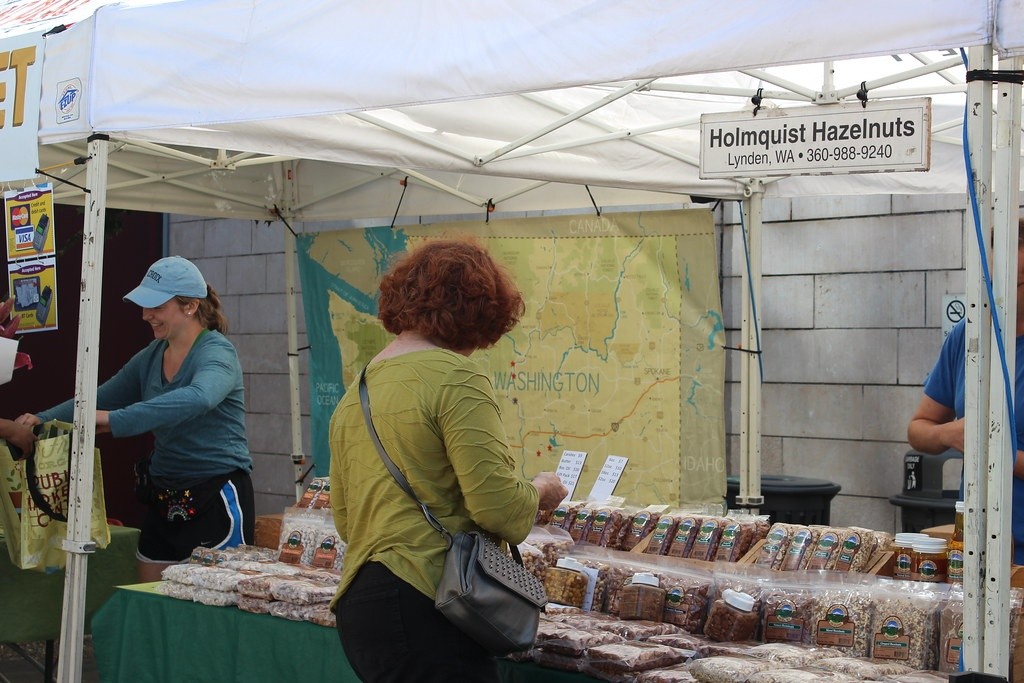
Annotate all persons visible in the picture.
[15,255,254,583]
[907,218,1024,567]
[0,418,39,459]
[328,238,569,683]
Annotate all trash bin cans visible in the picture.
[727,474,844,528]
[888,446,966,532]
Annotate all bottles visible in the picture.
[910,538,949,583]
[892,532,931,578]
[620,573,665,621]
[544,558,588,609]
[704,591,760,642]
[947,501,963,583]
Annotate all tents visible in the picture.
[0,0,1024,683]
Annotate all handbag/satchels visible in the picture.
[144,476,230,528]
[434,528,548,658]
[0,419,111,575]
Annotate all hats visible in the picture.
[122,255,207,308]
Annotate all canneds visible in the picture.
[892,533,947,583]
